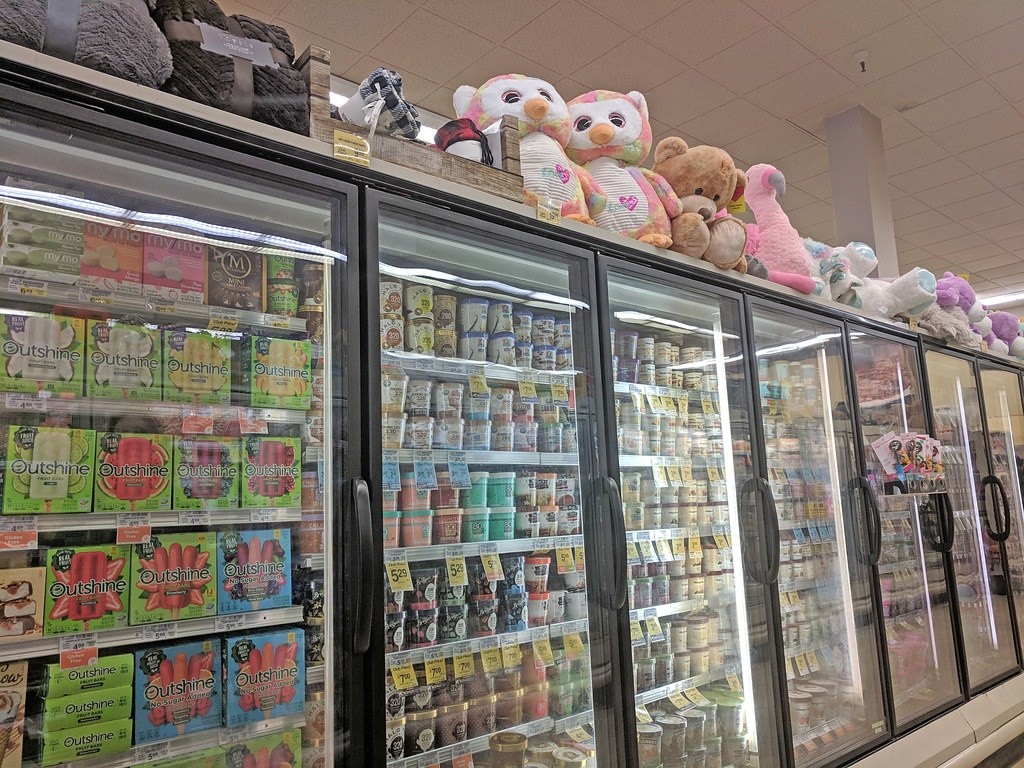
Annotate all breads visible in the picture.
[146,257,183,282]
[81,244,119,271]
[5,249,46,266]
[11,206,45,221]
[11,227,46,243]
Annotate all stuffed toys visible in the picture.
[452,73,606,227]
[745,164,1024,358]
[651,136,747,274]
[565,90,683,248]
